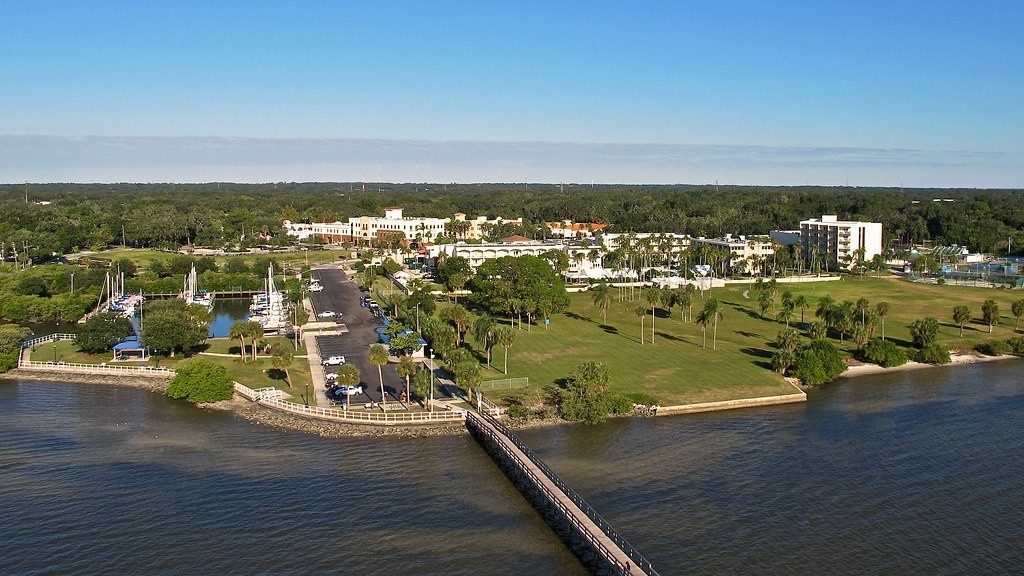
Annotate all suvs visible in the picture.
[323,355,345,367]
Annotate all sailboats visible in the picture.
[76,262,146,327]
[174,261,216,319]
[248,261,286,335]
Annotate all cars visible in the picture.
[307,281,323,293]
[368,300,378,308]
[364,296,373,303]
[318,310,336,319]
[323,372,363,399]
